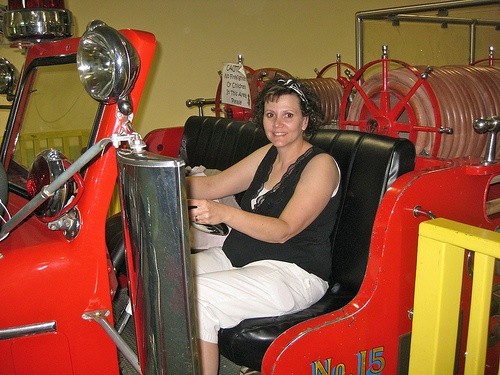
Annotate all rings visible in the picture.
[196,216,198,223]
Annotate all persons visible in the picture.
[185,76,343,375]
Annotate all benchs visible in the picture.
[106,116,417,371]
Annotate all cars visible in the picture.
[0,0,500,375]
